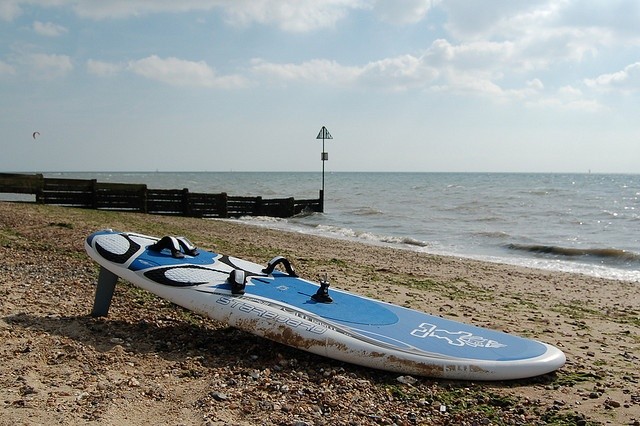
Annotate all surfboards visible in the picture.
[84,228,566,381]
